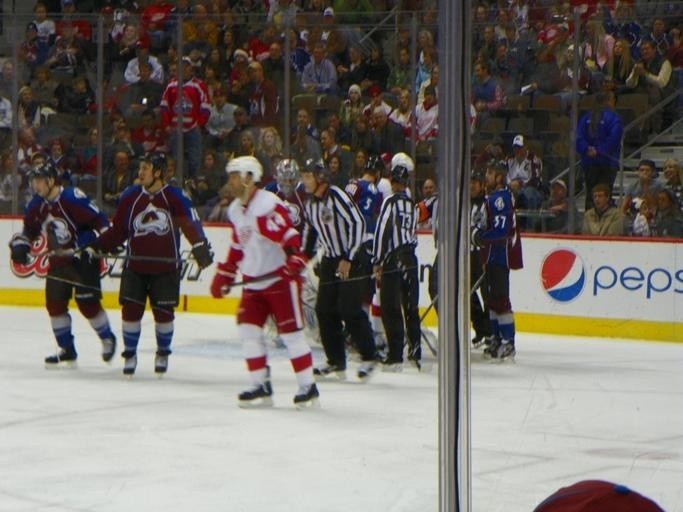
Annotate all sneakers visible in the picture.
[122,349,136,374]
[154,349,172,372]
[314,360,346,376]
[356,358,379,378]
[473,333,515,359]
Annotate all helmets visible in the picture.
[301,157,331,183]
[226,155,264,183]
[27,162,57,179]
[141,149,168,180]
[274,158,301,196]
[362,151,415,185]
[470,157,508,181]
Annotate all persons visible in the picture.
[8,160,115,366]
[469,290,478,293]
[0,1,683,243]
[209,156,322,412]
[76,152,215,375]
[260,150,518,383]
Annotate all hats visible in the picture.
[424,87,434,97]
[349,83,377,96]
[325,8,333,15]
[133,40,263,97]
[26,23,36,31]
[511,133,527,147]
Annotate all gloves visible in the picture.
[71,244,99,273]
[192,241,214,268]
[213,262,236,299]
[274,252,309,282]
[10,234,31,265]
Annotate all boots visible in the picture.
[294,384,319,404]
[45,337,76,364]
[239,365,272,400]
[381,339,421,364]
[101,334,115,360]
